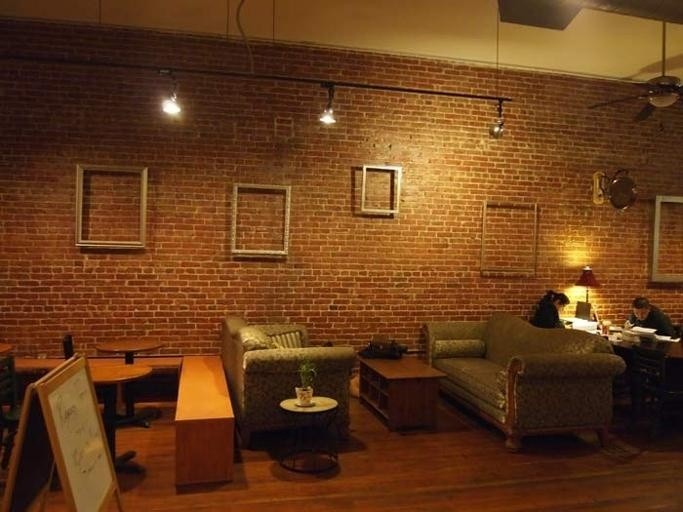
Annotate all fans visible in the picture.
[588,21,683,123]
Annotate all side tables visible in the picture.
[279,397,338,475]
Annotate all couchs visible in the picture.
[422,311,626,451]
[225,316,355,444]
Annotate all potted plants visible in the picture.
[295,354,318,404]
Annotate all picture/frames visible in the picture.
[74,164,149,249]
[479,200,539,279]
[230,183,291,258]
[360,164,402,216]
[651,195,683,283]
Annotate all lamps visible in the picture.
[592,170,637,210]
[488,100,505,140]
[162,77,182,116]
[318,86,337,125]
[575,265,601,303]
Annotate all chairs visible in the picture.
[61,336,78,357]
[631,348,683,439]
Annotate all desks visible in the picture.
[610,328,682,363]
[96,339,165,428]
[88,364,152,475]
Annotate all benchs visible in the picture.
[14,358,180,375]
[175,354,235,487]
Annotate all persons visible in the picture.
[624,297,674,337]
[533,291,569,328]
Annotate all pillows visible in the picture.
[239,327,272,350]
[434,339,485,355]
[268,330,305,347]
[225,316,244,336]
